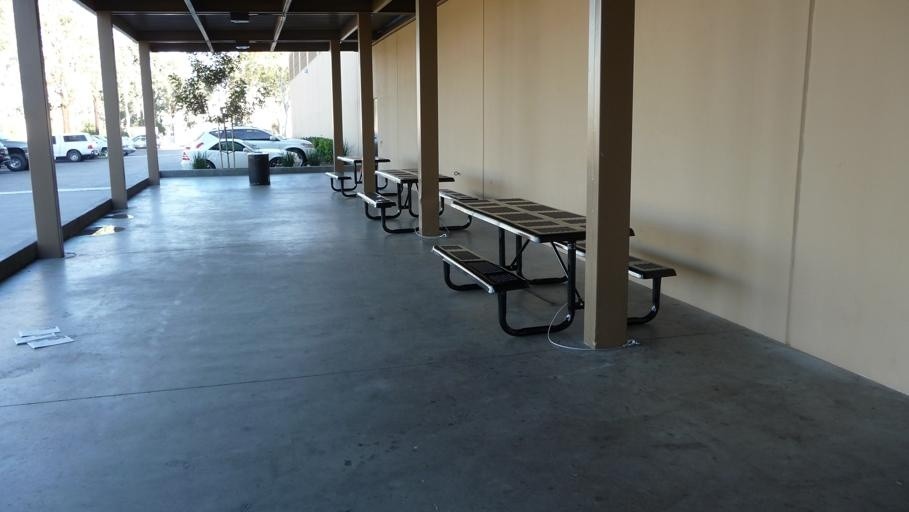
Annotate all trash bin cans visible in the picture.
[247,151,270,186]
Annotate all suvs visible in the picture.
[188,126,319,167]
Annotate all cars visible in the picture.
[182,140,301,169]
[0,134,29,171]
[92,134,136,156]
[131,135,160,149]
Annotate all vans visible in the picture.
[50,133,99,162]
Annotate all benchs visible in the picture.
[409,189,479,231]
[432,243,576,334]
[325,172,357,197]
[517,238,677,324]
[356,192,419,235]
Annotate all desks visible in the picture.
[443,197,661,338]
[331,155,403,197]
[365,169,472,234]
